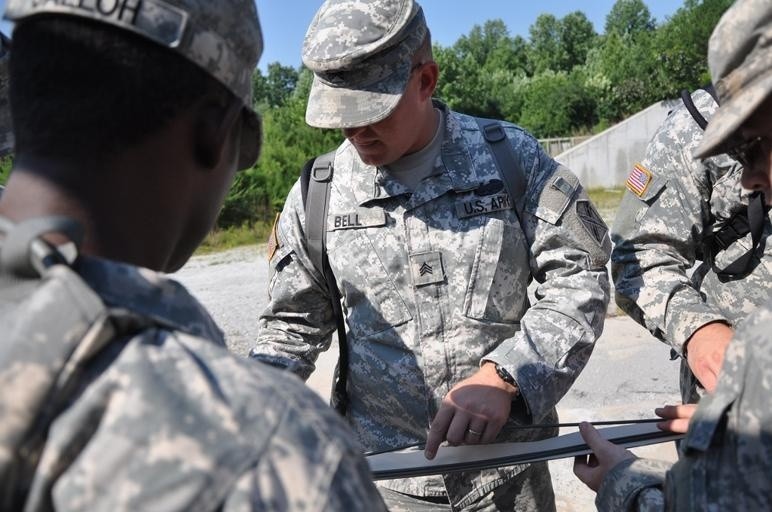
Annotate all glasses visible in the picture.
[723,131,772,166]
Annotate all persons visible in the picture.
[567,1,771,510]
[245,0,611,512]
[609,79,772,456]
[0,0,392,512]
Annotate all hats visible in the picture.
[2,0,265,171]
[693,0,771,158]
[299,0,429,131]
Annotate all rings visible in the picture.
[467,428,482,436]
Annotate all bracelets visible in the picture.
[493,363,516,387]
